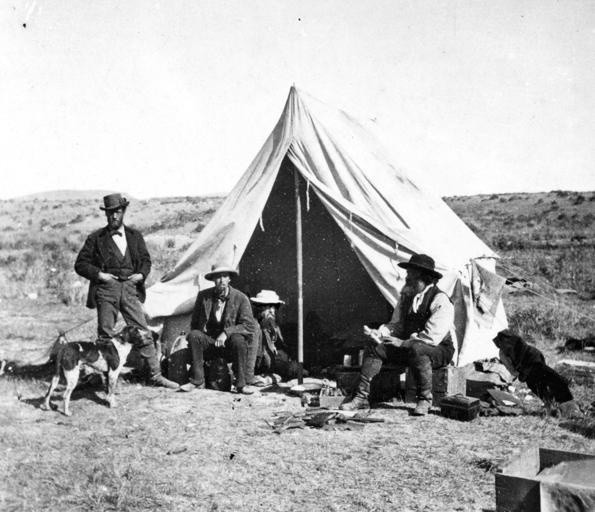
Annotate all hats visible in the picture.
[249,289,286,305]
[204,262,240,281]
[99,193,129,210]
[397,252,444,279]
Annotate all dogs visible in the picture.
[42,324,152,417]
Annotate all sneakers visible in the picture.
[180,382,206,391]
[145,372,180,389]
[237,384,255,394]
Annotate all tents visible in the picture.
[137,86,508,371]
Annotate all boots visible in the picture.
[413,361,433,416]
[339,356,384,411]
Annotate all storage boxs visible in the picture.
[489,439,595,511]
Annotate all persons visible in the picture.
[340,255,456,416]
[179,263,255,395]
[75,193,180,389]
[249,289,310,380]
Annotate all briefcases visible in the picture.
[439,392,481,422]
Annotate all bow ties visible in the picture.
[212,294,226,302]
[109,230,122,237]
